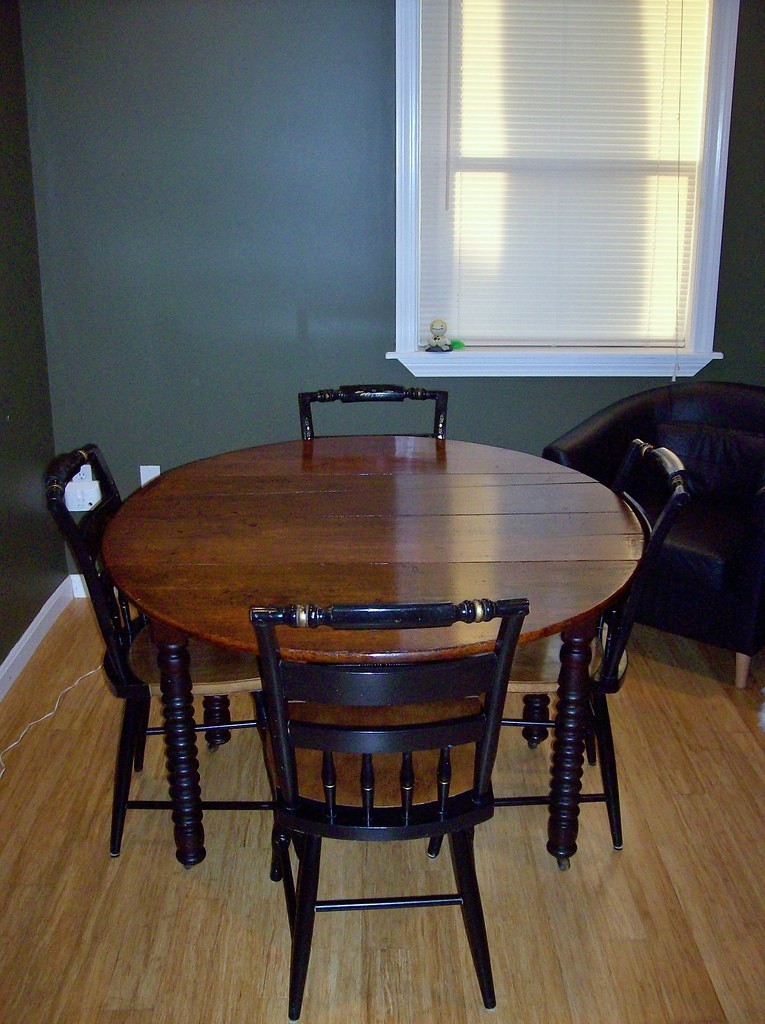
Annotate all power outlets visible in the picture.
[71,462,93,481]
[140,464,161,488]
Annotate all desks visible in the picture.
[98,431,644,876]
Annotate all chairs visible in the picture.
[37,440,281,855]
[295,382,452,442]
[250,590,530,1020]
[491,431,686,850]
[541,380,765,689]
